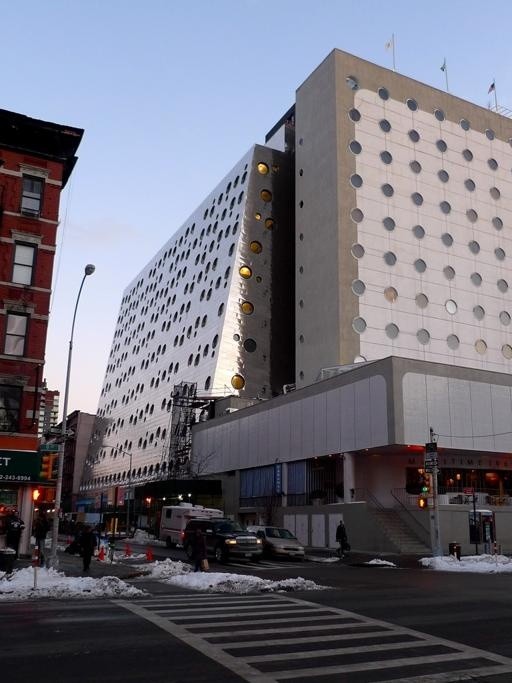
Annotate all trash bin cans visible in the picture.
[448,541,461,561]
[0,548,16,574]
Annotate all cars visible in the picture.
[246,525,305,561]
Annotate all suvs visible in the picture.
[182,517,264,562]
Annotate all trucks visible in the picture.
[159,505,231,549]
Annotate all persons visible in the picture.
[191,529,207,572]
[2,511,97,572]
[336,520,348,554]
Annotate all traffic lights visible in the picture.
[39,453,59,482]
[418,468,434,509]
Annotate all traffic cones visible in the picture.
[32,546,40,567]
[98,544,105,561]
[125,541,132,556]
[146,545,153,562]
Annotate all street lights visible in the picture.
[47,262,95,564]
[100,443,132,537]
